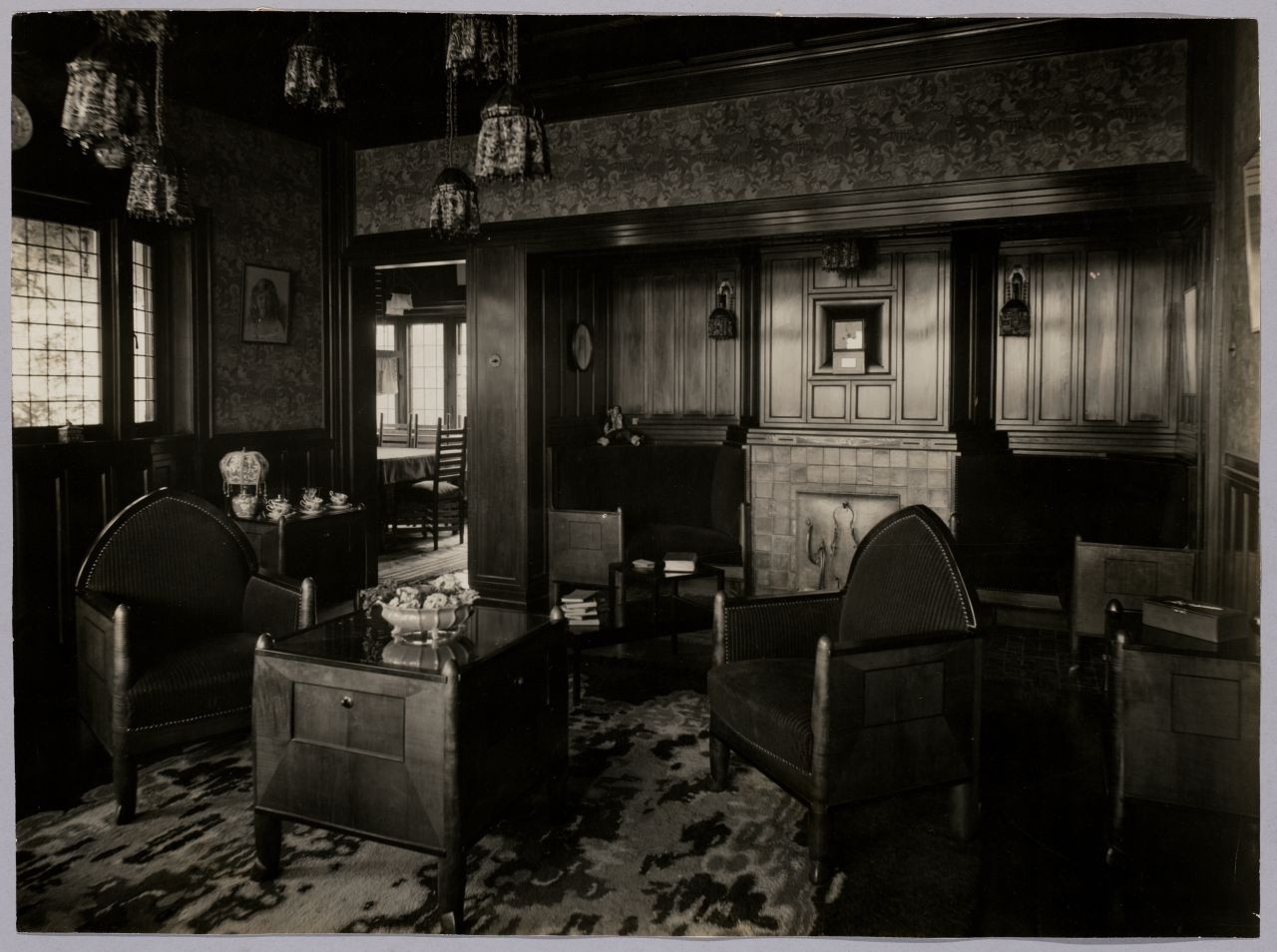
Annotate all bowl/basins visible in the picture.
[381,633,468,672]
[374,584,475,641]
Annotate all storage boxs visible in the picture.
[1142,596,1250,644]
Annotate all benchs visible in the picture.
[541,441,742,619]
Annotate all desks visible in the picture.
[377,447,468,504]
[1071,536,1205,654]
[1108,598,1260,830]
[252,603,569,935]
[227,503,378,589]
[608,562,725,629]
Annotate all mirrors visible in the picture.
[1242,146,1261,335]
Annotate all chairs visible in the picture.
[74,486,316,825]
[708,505,979,883]
[379,413,468,551]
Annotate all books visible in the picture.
[1141,596,1249,643]
[561,590,610,626]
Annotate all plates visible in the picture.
[267,513,292,520]
[326,503,352,510]
[300,510,325,515]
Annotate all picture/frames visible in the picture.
[241,262,295,345]
[571,323,593,371]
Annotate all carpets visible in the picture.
[16,690,824,936]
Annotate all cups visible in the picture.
[267,498,292,513]
[328,490,348,506]
[301,495,323,511]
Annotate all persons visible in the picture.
[243,279,284,341]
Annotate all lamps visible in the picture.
[278,11,346,112]
[59,10,197,226]
[218,448,269,519]
[708,294,735,339]
[822,237,862,271]
[999,283,1031,338]
[429,14,550,245]
